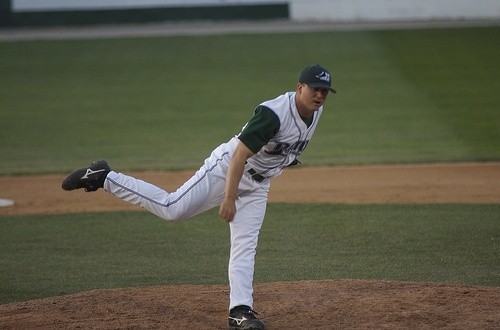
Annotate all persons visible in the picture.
[62,64,337,330]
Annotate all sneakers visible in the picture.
[227,305,264,330]
[62,160,111,192]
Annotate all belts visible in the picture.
[245,161,264,182]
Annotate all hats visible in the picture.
[299,64,337,93]
